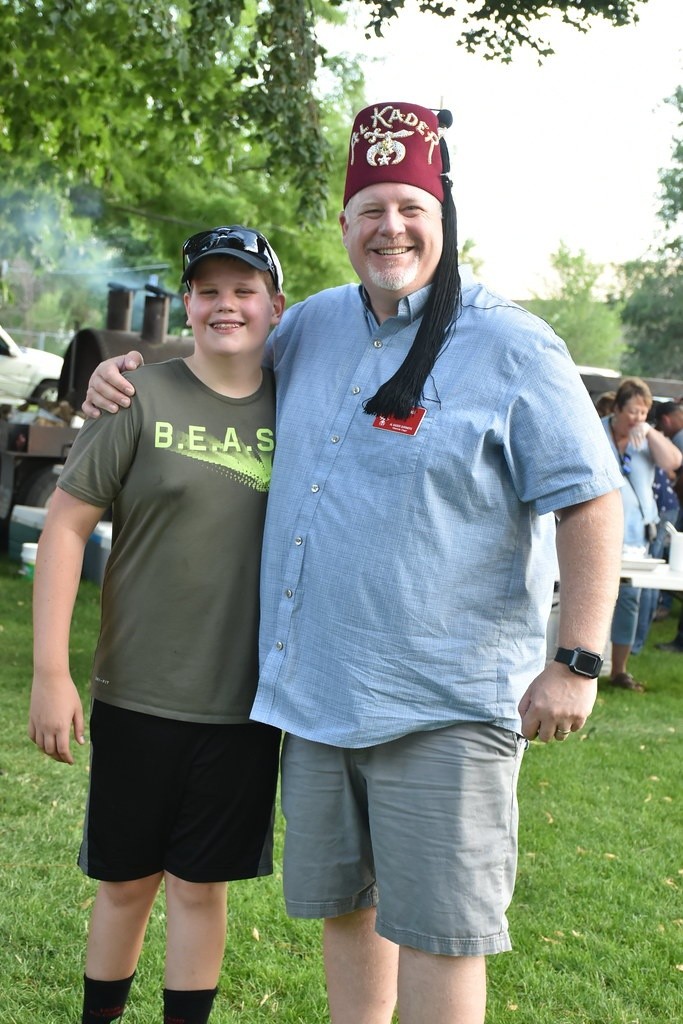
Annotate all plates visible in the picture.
[621,557,666,570]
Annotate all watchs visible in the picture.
[552,645,603,681]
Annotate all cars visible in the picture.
[0,324,68,407]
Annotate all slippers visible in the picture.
[612,673,643,692]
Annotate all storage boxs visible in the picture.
[8,504,46,562]
[82,521,113,582]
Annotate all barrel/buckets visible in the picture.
[20,542,38,582]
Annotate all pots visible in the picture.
[665,521,683,572]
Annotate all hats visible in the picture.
[342,101,445,206]
[181,226,288,293]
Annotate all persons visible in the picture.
[28,224,289,1019]
[595,385,681,550]
[76,99,629,1024]
[600,379,683,689]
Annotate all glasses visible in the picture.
[622,451,632,477]
[181,228,278,289]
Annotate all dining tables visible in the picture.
[616,564,683,592]
